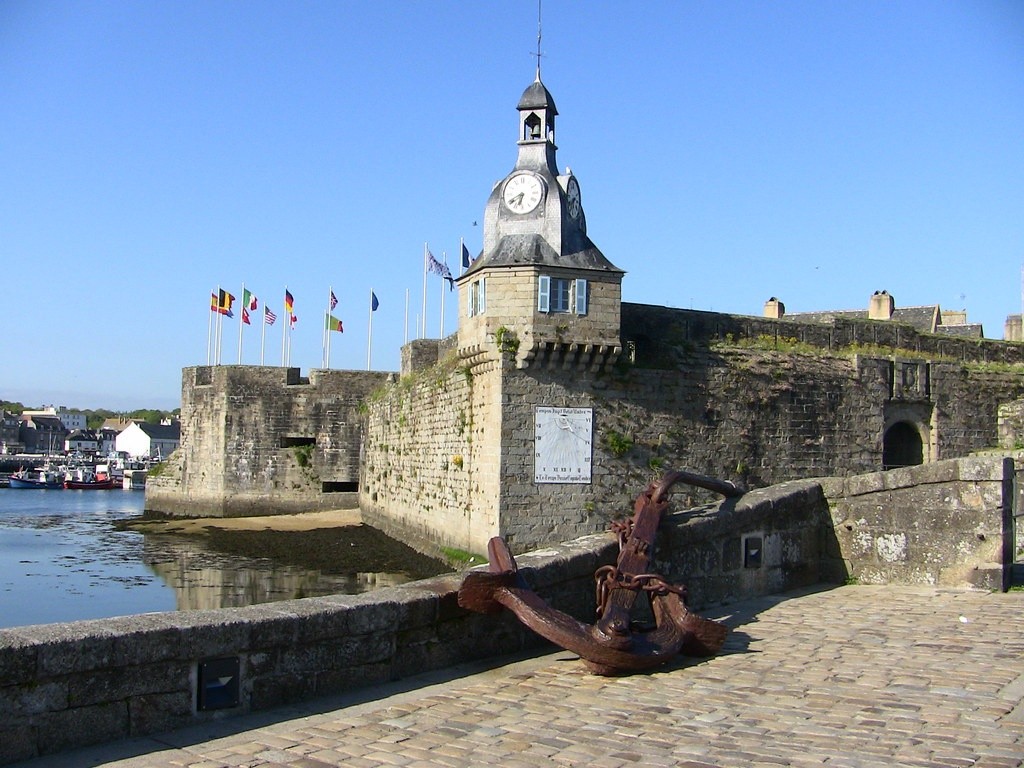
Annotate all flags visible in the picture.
[462,242,476,267]
[324,290,344,334]
[425,248,455,293]
[286,289,299,330]
[263,305,277,326]
[243,287,258,326]
[210,287,236,320]
[372,292,379,312]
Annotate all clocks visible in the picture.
[568,180,580,218]
[504,175,542,214]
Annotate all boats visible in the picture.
[5,429,125,489]
